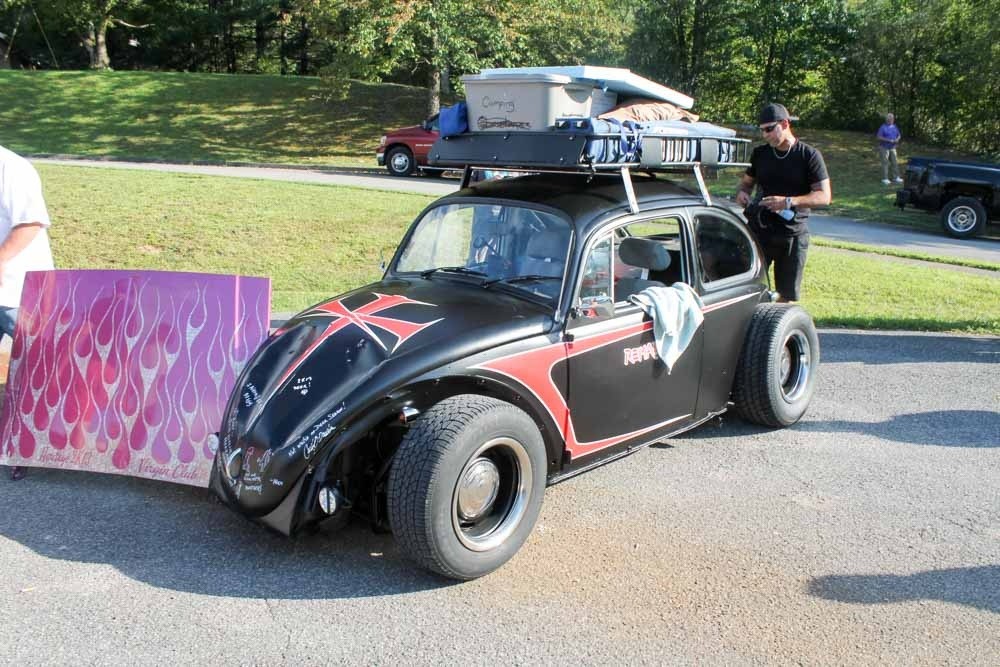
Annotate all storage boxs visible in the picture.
[460,73,619,131]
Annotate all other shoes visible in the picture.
[892,176,904,183]
[881,178,892,185]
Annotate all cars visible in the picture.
[209,166,821,581]
[375,112,445,179]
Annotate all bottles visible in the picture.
[762,197,794,220]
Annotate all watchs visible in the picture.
[785,197,792,210]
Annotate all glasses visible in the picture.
[758,122,779,134]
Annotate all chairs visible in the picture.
[614,237,671,301]
[518,229,564,296]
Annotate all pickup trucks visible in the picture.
[894,155,1000,240]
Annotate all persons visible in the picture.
[0,142,56,338]
[736,105,832,301]
[876,113,904,184]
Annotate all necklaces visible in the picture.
[773,137,795,159]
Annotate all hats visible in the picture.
[759,103,792,123]
